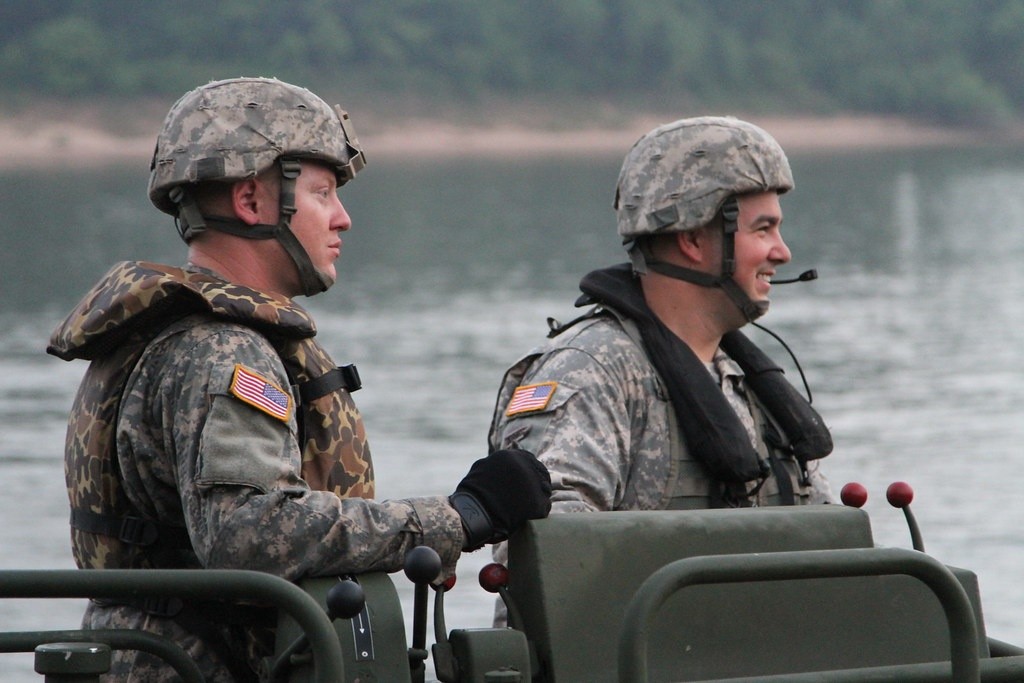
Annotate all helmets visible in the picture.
[147,77,367,215]
[612,116,796,235]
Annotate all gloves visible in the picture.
[448,449,553,552]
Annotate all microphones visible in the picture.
[770,268,818,284]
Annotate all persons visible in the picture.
[487,116,838,632]
[46,78,552,683]
